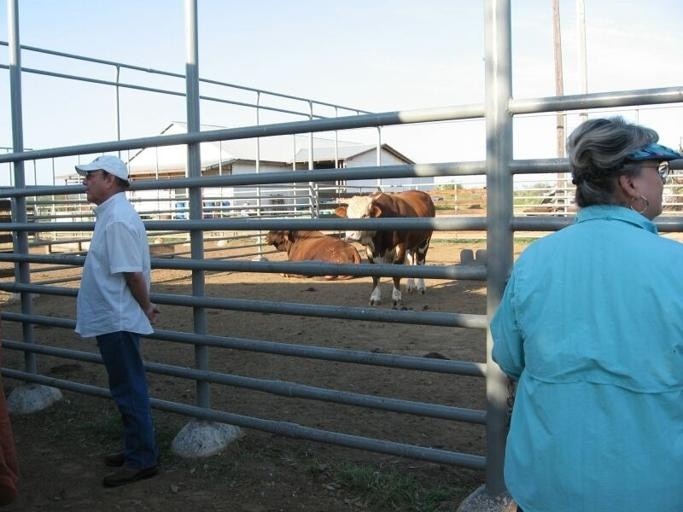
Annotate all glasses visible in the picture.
[85,171,103,179]
[636,163,672,176]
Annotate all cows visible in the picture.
[334,189,437,308]
[263,229,362,281]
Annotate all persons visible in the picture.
[487,115,682,512]
[73,155,161,488]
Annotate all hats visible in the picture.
[74,156,129,182]
[610,142,683,170]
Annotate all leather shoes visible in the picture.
[104,451,164,487]
